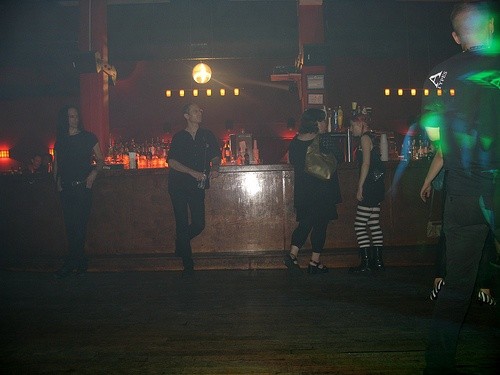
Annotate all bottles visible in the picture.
[409,135,428,161]
[224,143,249,166]
[322,104,343,134]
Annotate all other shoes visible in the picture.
[430,279,447,304]
[476,291,500,308]
[183,266,194,282]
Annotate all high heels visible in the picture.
[284,253,301,272]
[308,259,328,273]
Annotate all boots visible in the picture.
[358,247,369,272]
[372,246,385,272]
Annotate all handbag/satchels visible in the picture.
[304,135,338,183]
[367,163,386,183]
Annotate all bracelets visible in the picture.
[94,168,99,172]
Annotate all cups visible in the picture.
[128,152,137,169]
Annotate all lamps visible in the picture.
[192,60,212,84]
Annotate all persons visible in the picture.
[53,104,105,278]
[420,0,500,375]
[284,109,341,273]
[164,101,220,279]
[23,155,43,174]
[346,114,386,272]
